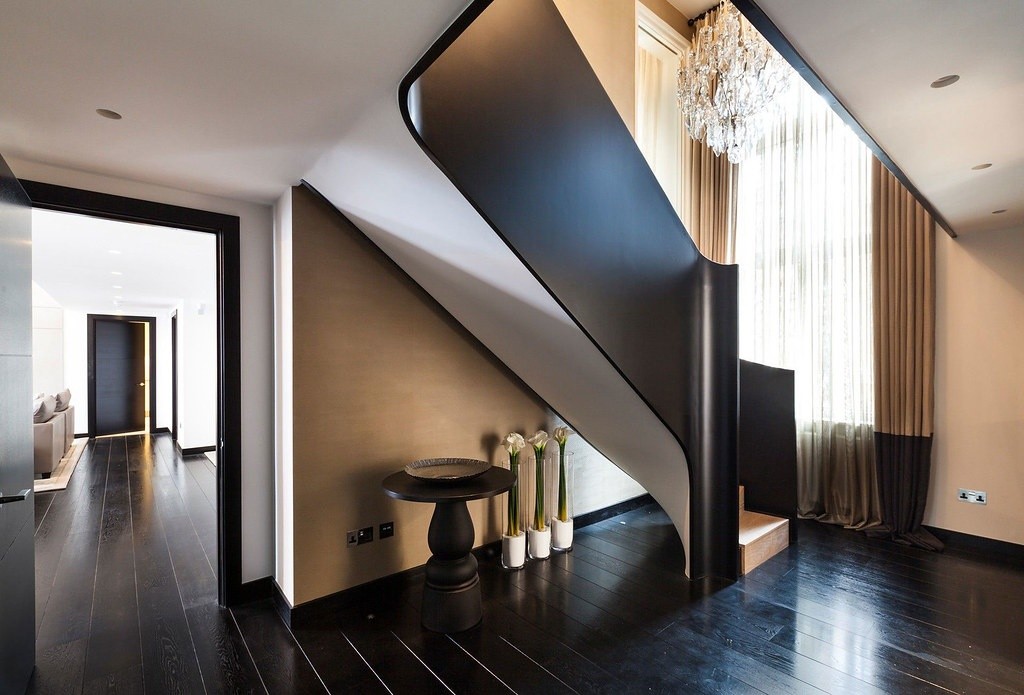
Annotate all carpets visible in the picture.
[34,437,89,493]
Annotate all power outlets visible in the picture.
[346,526,373,549]
[379,521,394,540]
[958,488,987,505]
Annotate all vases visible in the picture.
[501,458,526,570]
[550,451,574,552]
[527,454,551,560]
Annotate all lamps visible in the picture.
[676,0,798,165]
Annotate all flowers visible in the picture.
[526,430,553,531]
[552,426,576,522]
[501,432,526,535]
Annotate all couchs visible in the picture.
[34,388,75,479]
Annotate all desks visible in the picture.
[380,467,516,634]
